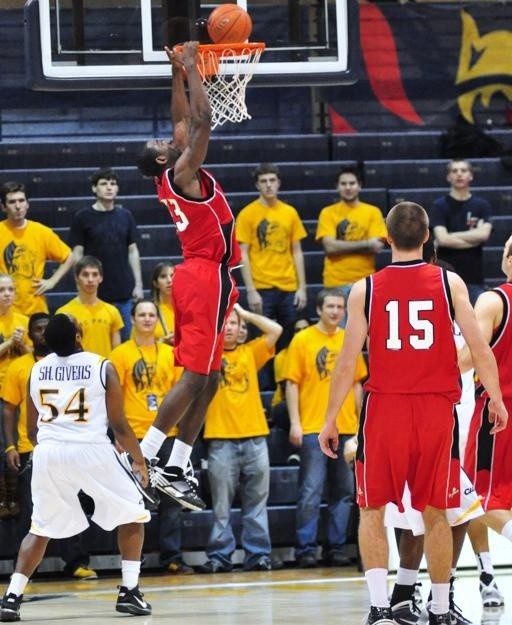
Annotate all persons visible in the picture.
[0,180,74,316]
[279,286,369,568]
[129,259,175,346]
[0,313,153,623]
[390,259,476,624]
[318,201,509,625]
[316,167,389,329]
[430,158,494,307]
[0,312,99,583]
[107,295,195,577]
[0,275,30,468]
[235,162,309,352]
[457,235,512,607]
[198,301,284,572]
[74,168,144,342]
[56,255,125,357]
[236,318,248,343]
[269,315,311,464]
[120,39,243,512]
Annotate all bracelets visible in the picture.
[5,443,16,455]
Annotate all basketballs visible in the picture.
[206,2,253,44]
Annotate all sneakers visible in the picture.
[0,592,23,622]
[72,566,97,579]
[322,551,351,566]
[286,451,301,465]
[390,596,421,625]
[368,605,394,625]
[194,561,232,573]
[244,557,273,570]
[164,561,195,574]
[121,450,161,506]
[296,552,318,567]
[479,571,504,606]
[429,610,472,625]
[116,584,151,615]
[153,460,208,514]
